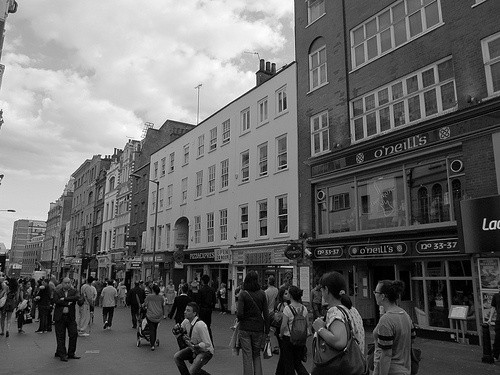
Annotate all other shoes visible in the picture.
[272,350,279,354]
[133,325,137,328]
[35,329,47,333]
[60,356,68,362]
[104,321,108,329]
[108,327,111,330]
[6,331,9,337]
[67,355,81,359]
[78,332,89,336]
[0,333,4,335]
[150,346,155,350]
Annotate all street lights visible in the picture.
[38,232,54,280]
[129,174,160,281]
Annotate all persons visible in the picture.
[53,277,81,362]
[311,282,322,318]
[127,275,218,351]
[0,272,126,337]
[488,281,500,362]
[312,272,365,354]
[317,327,323,333]
[232,270,309,375]
[217,282,228,315]
[174,302,214,375]
[369,281,416,375]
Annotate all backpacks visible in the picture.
[287,305,307,345]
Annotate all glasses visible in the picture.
[373,291,387,297]
[316,287,323,292]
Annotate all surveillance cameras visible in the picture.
[305,249,312,257]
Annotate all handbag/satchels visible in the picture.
[0,293,7,307]
[23,313,32,324]
[302,347,307,362]
[411,348,422,375]
[77,294,85,306]
[264,341,273,360]
[269,312,283,328]
[312,305,369,375]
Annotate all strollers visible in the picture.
[136,312,160,348]
